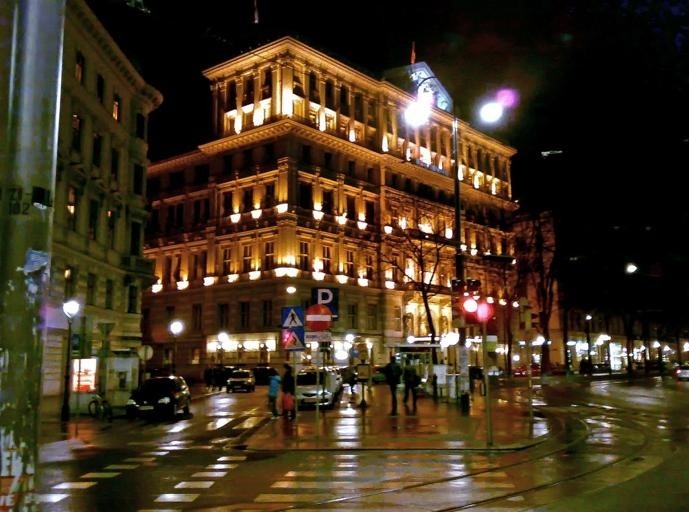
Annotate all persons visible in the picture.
[401,359,418,409]
[278,363,296,422]
[267,366,284,420]
[377,355,401,416]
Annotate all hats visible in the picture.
[674,365,689,380]
[466,362,567,379]
[204,364,386,410]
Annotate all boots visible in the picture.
[126,376,192,423]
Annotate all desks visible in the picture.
[218,331,229,364]
[60,299,81,422]
[170,320,184,374]
[404,75,472,417]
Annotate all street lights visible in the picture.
[284,395,293,410]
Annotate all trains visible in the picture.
[305,304,332,331]
